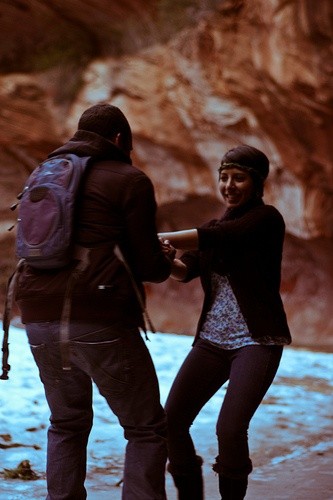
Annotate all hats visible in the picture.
[221,144,270,180]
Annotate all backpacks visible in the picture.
[16,152,92,271]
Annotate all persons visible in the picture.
[158,143,292,500]
[16,103,177,500]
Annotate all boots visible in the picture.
[212,459,252,500]
[168,455,204,500]
